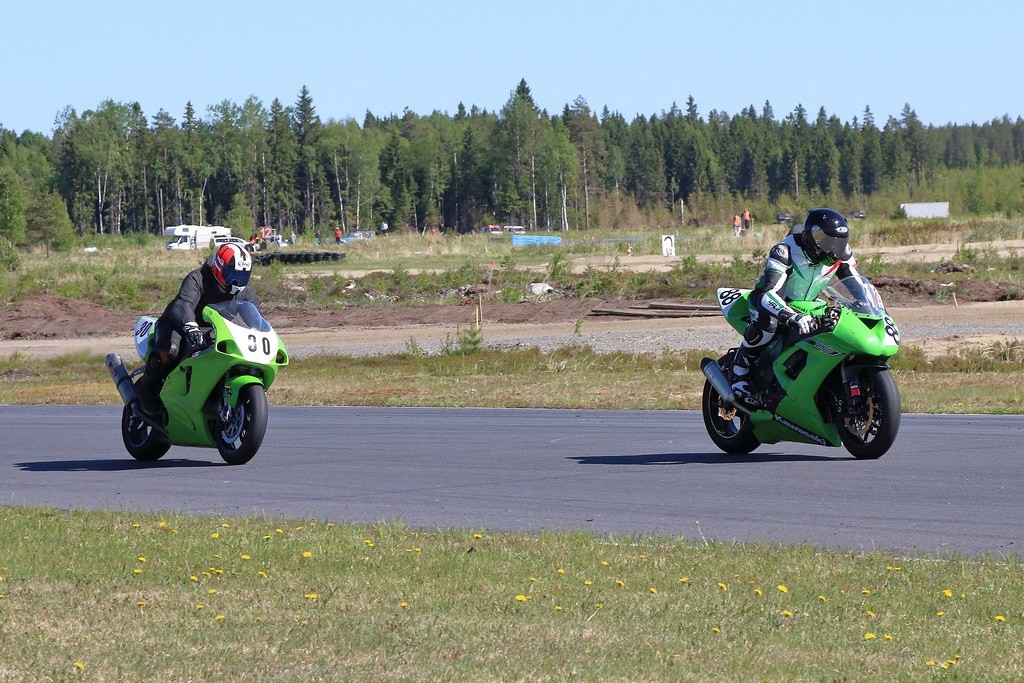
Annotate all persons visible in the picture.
[428,224,445,239]
[381,221,388,238]
[315,229,322,245]
[728,208,877,397]
[134,243,253,416]
[334,227,342,243]
[289,230,296,245]
[732,208,751,237]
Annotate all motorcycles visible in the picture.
[106,300,289,466]
[699,275,902,460]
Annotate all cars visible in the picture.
[850,209,866,219]
[264,235,289,248]
[211,237,260,252]
[340,232,371,243]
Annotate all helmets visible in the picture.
[804,207,848,265]
[211,243,253,298]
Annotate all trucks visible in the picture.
[504,226,525,234]
[164,225,231,249]
[481,225,503,234]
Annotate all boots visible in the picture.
[134,356,162,415]
[732,339,759,398]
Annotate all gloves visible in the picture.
[777,306,815,334]
[183,321,204,350]
